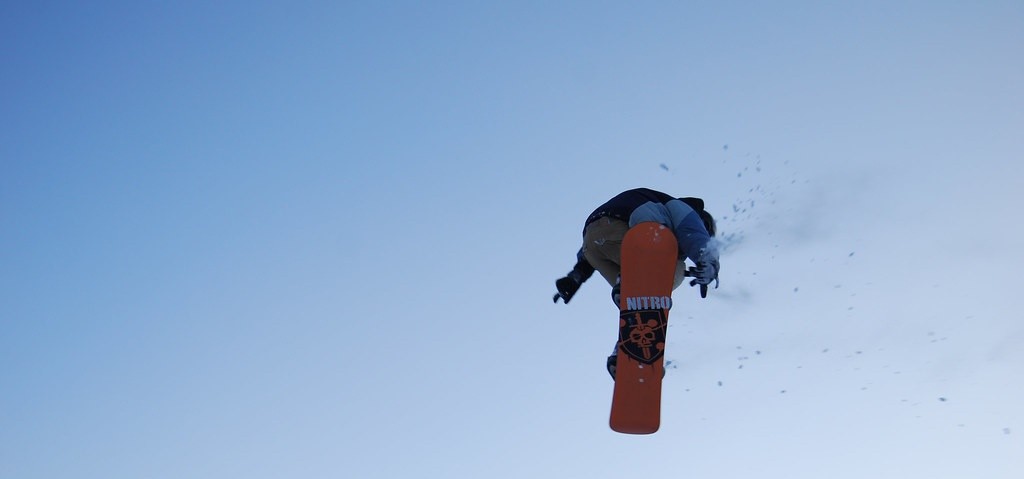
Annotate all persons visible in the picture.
[555,188,721,384]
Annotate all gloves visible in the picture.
[682,260,720,298]
[553,277,580,304]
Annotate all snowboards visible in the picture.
[610,219,678,434]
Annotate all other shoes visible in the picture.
[607,356,665,383]
[611,287,672,312]
